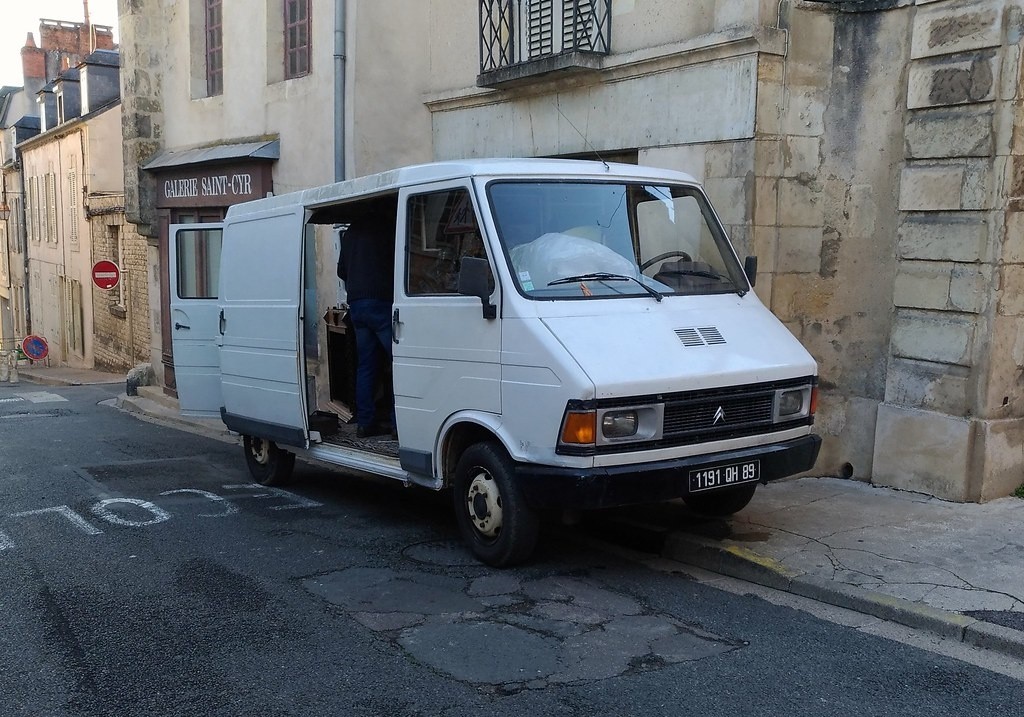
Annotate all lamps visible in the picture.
[0,191,26,221]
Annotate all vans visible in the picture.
[168,158,824,564]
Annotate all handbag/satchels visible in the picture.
[308,410,342,435]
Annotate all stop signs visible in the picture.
[92,260,120,290]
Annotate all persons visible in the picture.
[337,197,396,440]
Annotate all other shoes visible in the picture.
[357,424,391,437]
[391,428,398,440]
